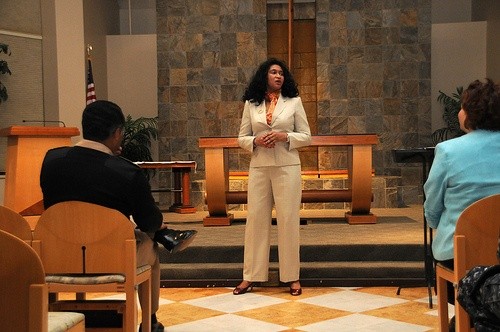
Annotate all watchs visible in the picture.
[285,133,289,142]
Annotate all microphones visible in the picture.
[22,120,65,128]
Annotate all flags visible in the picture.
[86,58,96,105]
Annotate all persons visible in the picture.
[233,57,312,296]
[456,245,500,332]
[41,98,197,332]
[422,78,500,304]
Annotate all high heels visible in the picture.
[233,282,253,295]
[290,281,302,296]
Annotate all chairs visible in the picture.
[0,204,33,258]
[436,194,500,332]
[0,230,85,332]
[36,204,152,332]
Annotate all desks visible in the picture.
[393,149,443,309]
[198,134,379,225]
[135,161,197,213]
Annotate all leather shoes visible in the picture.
[161,230,198,253]
[140,322,164,332]
